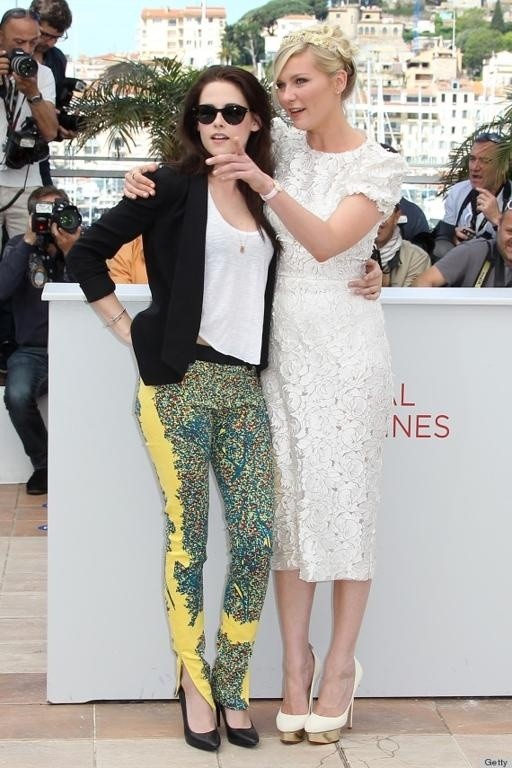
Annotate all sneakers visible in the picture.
[26,469,47,495]
[1,339,16,374]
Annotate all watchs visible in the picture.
[27,93,42,105]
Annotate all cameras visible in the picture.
[15,117,50,163]
[31,198,81,243]
[55,77,88,142]
[3,49,38,78]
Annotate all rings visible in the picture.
[132,173,135,179]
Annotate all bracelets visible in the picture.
[492,226,497,231]
[104,307,127,330]
[258,179,283,202]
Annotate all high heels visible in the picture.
[276,643,321,744]
[304,656,364,744]
[214,697,259,747]
[178,685,221,751]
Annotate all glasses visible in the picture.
[504,200,512,211]
[478,133,502,143]
[40,31,68,43]
[190,103,250,125]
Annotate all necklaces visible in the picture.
[236,219,249,253]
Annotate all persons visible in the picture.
[119,22,409,744]
[441,132,512,247]
[410,205,512,287]
[1,185,91,496]
[360,201,431,291]
[105,232,150,287]
[65,63,385,754]
[20,1,85,192]
[0,6,60,240]
[378,142,429,243]
